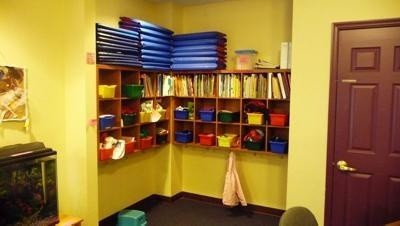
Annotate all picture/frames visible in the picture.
[1,64,28,122]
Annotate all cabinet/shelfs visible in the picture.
[96,64,291,163]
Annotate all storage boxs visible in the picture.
[235,48,258,70]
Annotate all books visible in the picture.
[138,71,291,100]
